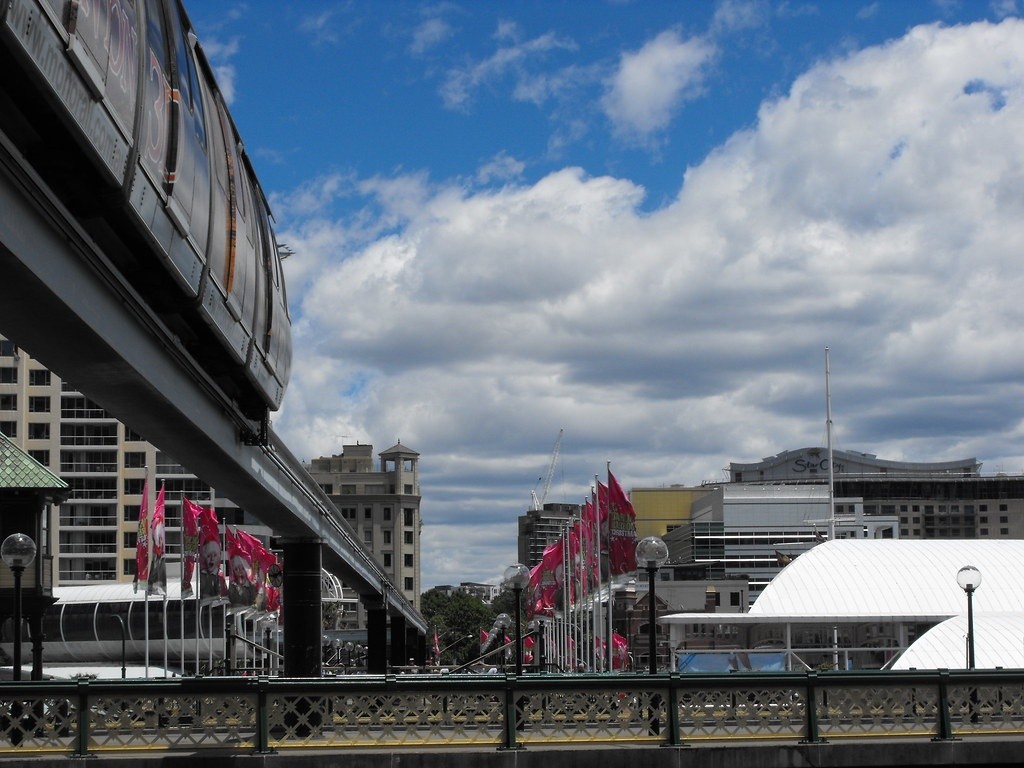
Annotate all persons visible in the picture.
[568,649,633,672]
[200,532,222,599]
[229,551,256,606]
[525,651,533,664]
[406,657,439,674]
[322,659,366,674]
[148,522,166,594]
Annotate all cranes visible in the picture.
[529,428,564,510]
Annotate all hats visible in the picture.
[202,532,220,562]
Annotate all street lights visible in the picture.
[481,613,511,674]
[504,563,530,731]
[635,536,669,737]
[1,533,36,748]
[322,635,368,667]
[957,565,982,723]
[260,616,277,676]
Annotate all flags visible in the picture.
[505,636,511,643]
[148,487,167,594]
[596,633,628,648]
[182,497,202,598]
[225,525,284,625]
[434,633,438,644]
[200,507,227,606]
[527,469,636,620]
[525,636,533,647]
[481,631,488,643]
[136,479,148,580]
[567,635,574,649]
